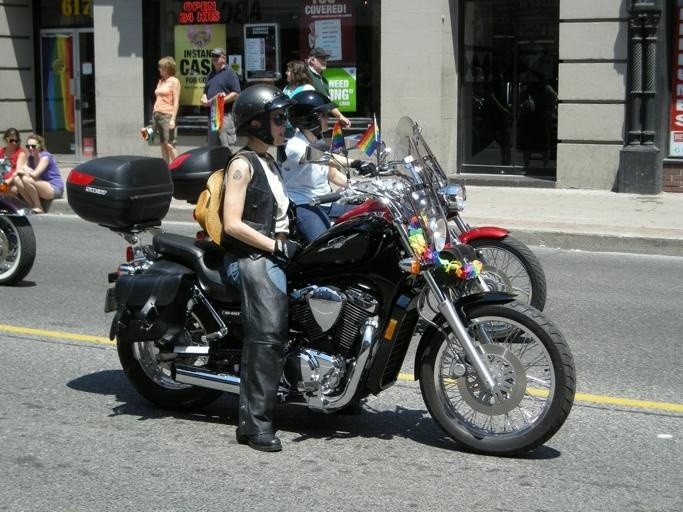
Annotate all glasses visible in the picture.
[269,112,288,127]
[25,143,41,150]
[6,137,21,144]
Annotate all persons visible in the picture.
[304,47,351,138]
[13,133,64,213]
[200,47,241,153]
[277,60,315,165]
[150,55,180,163]
[0,126,26,199]
[219,83,310,450]
[280,90,376,246]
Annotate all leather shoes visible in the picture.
[234,426,283,452]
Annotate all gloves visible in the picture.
[272,238,304,266]
[350,157,378,176]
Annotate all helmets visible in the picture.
[287,90,339,132]
[230,82,293,136]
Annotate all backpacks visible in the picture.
[193,154,253,249]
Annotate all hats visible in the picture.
[308,46,332,60]
[210,47,227,57]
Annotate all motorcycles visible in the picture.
[0,148,36,287]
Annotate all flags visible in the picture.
[209,93,223,131]
[357,122,380,156]
[329,120,343,155]
[40,34,75,134]
